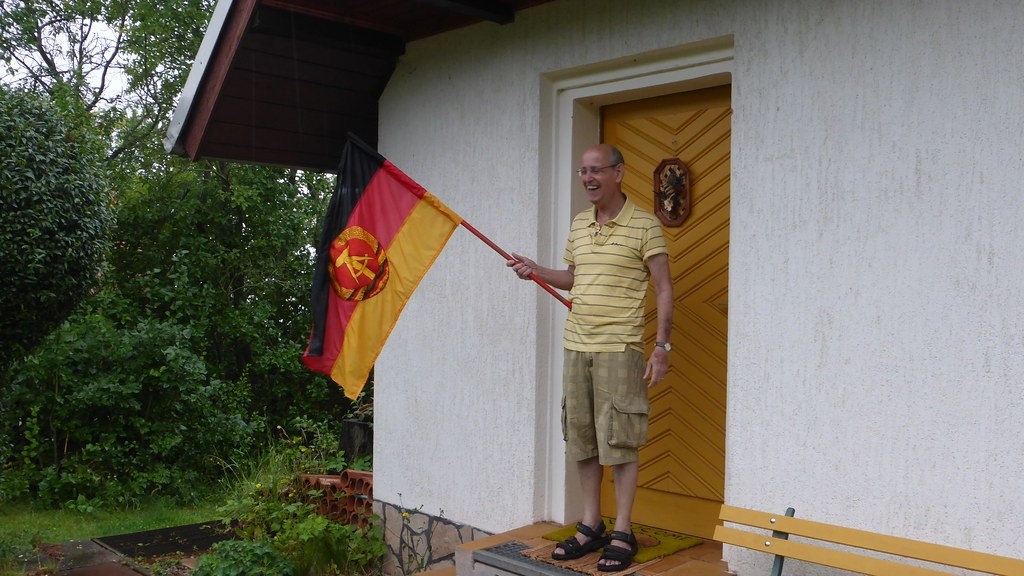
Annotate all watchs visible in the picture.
[656,342,672,352]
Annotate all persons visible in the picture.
[506,144,674,572]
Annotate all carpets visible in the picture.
[542,517,703,564]
[519,540,664,576]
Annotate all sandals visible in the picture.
[597,528,639,572]
[551,519,610,561]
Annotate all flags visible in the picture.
[302,134,464,400]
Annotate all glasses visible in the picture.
[577,164,617,177]
